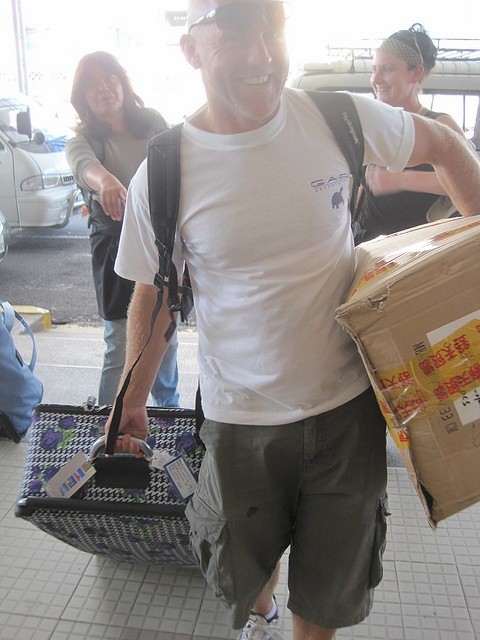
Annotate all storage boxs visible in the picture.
[335,214,479,529]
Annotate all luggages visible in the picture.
[14,403,204,568]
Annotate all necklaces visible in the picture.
[415,104,426,115]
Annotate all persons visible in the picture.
[68,52,180,416]
[355,22,474,245]
[103,0,479,640]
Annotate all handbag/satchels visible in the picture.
[0,302,43,445]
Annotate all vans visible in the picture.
[284,38,479,166]
[0,87,85,229]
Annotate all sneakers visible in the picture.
[236,594,281,640]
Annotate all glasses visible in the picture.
[184,0,293,33]
[386,30,424,65]
